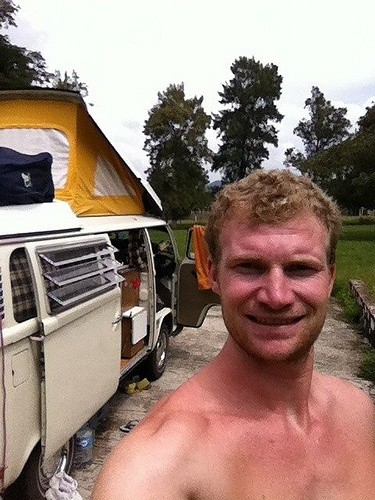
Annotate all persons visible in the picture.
[90,169,375,500]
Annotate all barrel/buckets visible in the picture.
[73,426,94,470]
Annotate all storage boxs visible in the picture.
[118,266,147,358]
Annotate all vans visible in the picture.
[0,199,223,500]
